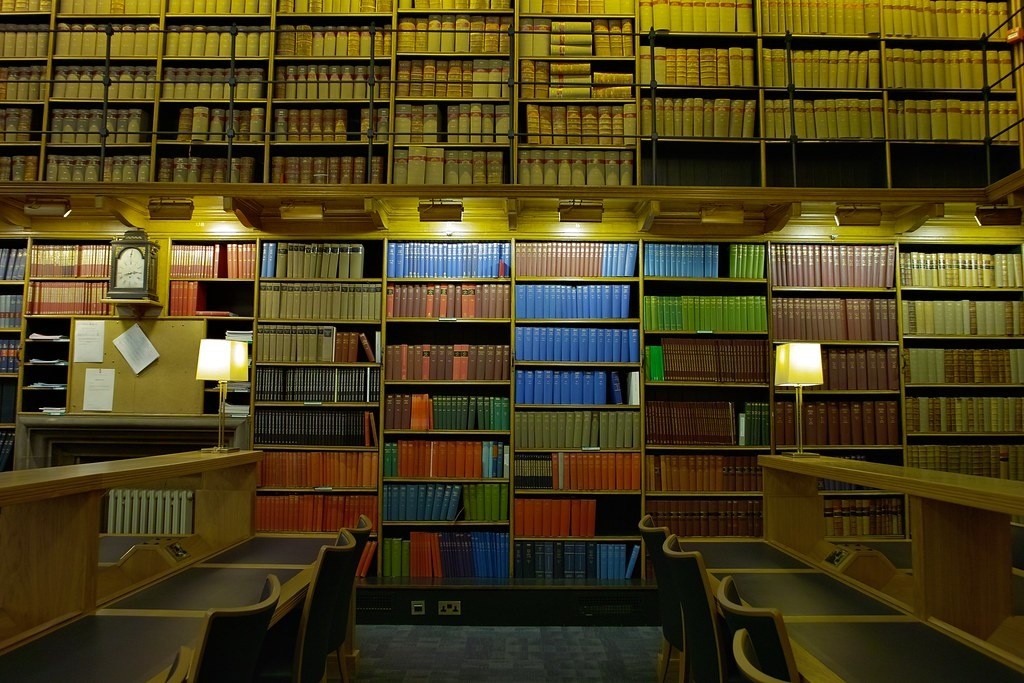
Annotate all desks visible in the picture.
[676,454,1024,683]
[0,450,338,683]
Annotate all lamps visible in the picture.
[148,197,195,220]
[775,342,824,458]
[23,196,72,218]
[279,201,326,220]
[834,204,883,226]
[699,203,744,223]
[558,201,604,223]
[974,206,1022,226]
[194,339,250,451]
[417,200,465,222]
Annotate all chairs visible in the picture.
[638,514,802,683]
[164,514,373,683]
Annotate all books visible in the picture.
[0,236,1024,581]
[2,0,1023,186]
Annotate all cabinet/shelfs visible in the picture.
[0,0,1024,628]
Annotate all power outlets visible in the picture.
[438,601,460,615]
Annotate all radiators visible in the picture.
[107,488,194,535]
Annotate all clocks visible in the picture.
[106,229,161,302]
